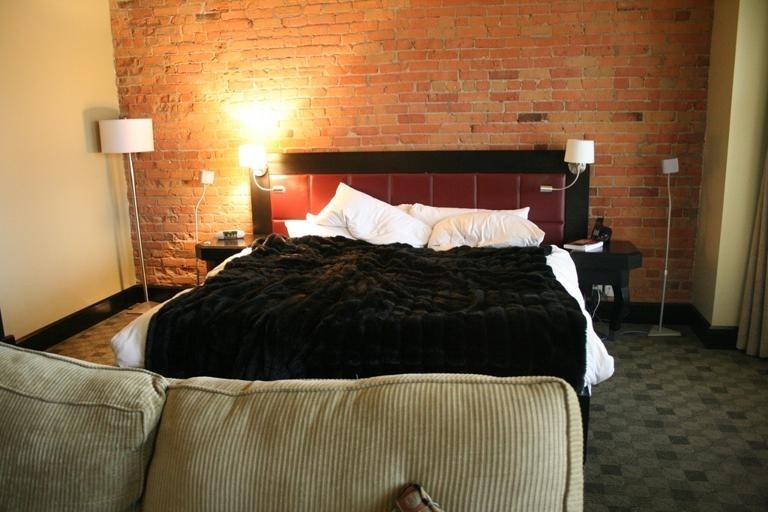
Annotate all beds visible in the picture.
[109,149,589,463]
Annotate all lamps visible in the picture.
[95,115,160,315]
[562,137,595,174]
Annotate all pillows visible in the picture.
[307,181,545,252]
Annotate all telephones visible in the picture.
[592,218,611,245]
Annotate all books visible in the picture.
[563,237,604,253]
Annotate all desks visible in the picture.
[194,234,256,275]
[570,239,642,331]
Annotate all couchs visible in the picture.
[0,342,584,512]
